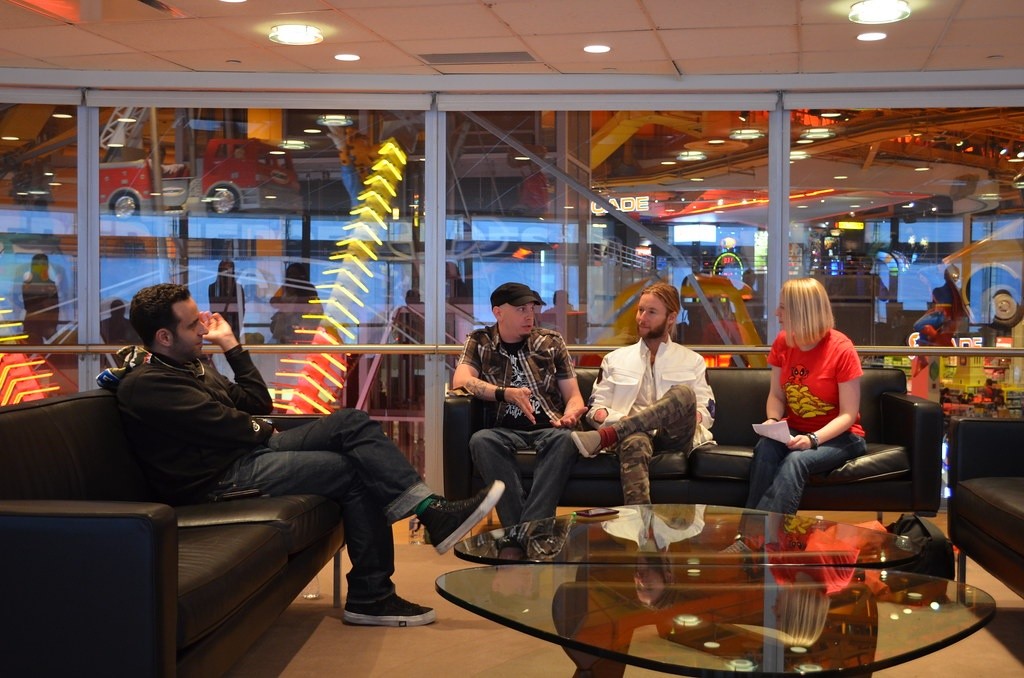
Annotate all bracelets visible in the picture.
[768,417,779,422]
[807,432,818,450]
[495,386,506,404]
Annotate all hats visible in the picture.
[490,282,541,308]
[532,290,546,305]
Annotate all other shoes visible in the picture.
[715,540,750,556]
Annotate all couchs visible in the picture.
[442,367,944,524]
[946,403,1024,600]
[0,388,347,678]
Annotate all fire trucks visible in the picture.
[95,136,301,223]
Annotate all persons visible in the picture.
[512,153,549,263]
[745,277,867,516]
[544,290,577,312]
[373,260,472,409]
[593,504,712,609]
[572,283,717,506]
[9,139,55,211]
[268,252,324,344]
[454,281,588,529]
[737,510,873,649]
[912,265,1024,346]
[681,254,766,349]
[844,253,891,302]
[99,300,143,345]
[21,253,60,345]
[108,283,506,629]
[208,258,245,343]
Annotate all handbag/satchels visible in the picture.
[879,512,955,577]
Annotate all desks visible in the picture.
[436,566,996,678]
[454,503,922,567]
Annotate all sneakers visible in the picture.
[344,593,435,627]
[571,431,601,458]
[421,481,505,555]
